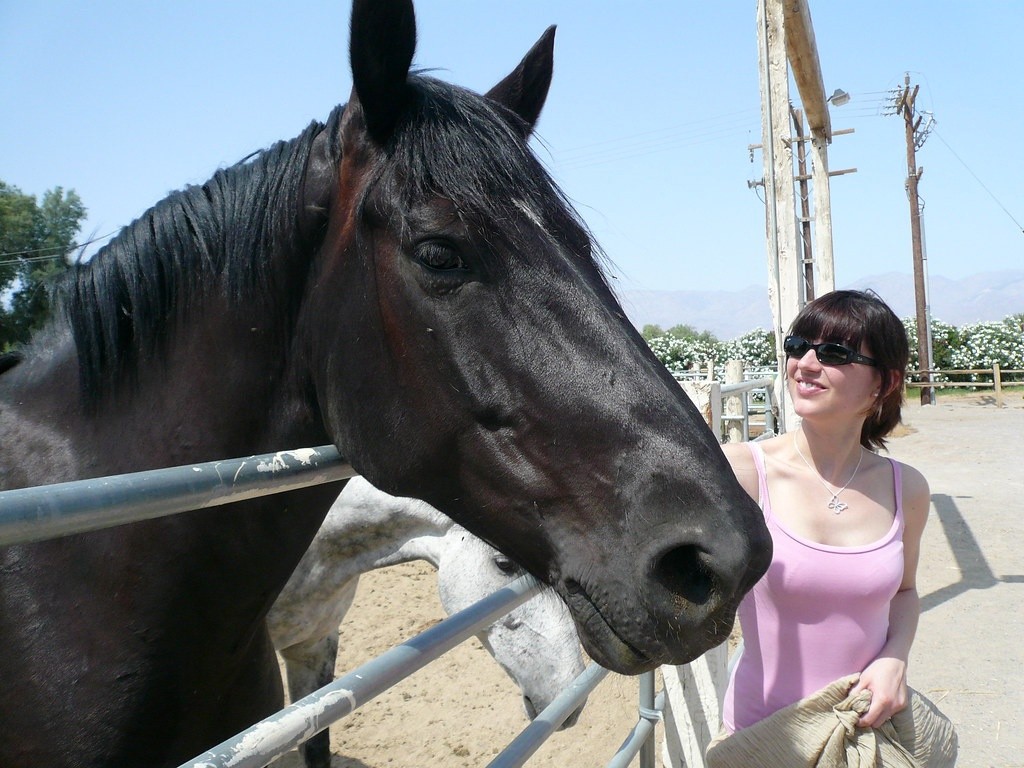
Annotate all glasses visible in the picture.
[784,335,885,369]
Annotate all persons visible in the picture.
[720,288,931,768]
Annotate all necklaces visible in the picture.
[794,427,864,514]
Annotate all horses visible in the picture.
[1,1,774,767]
